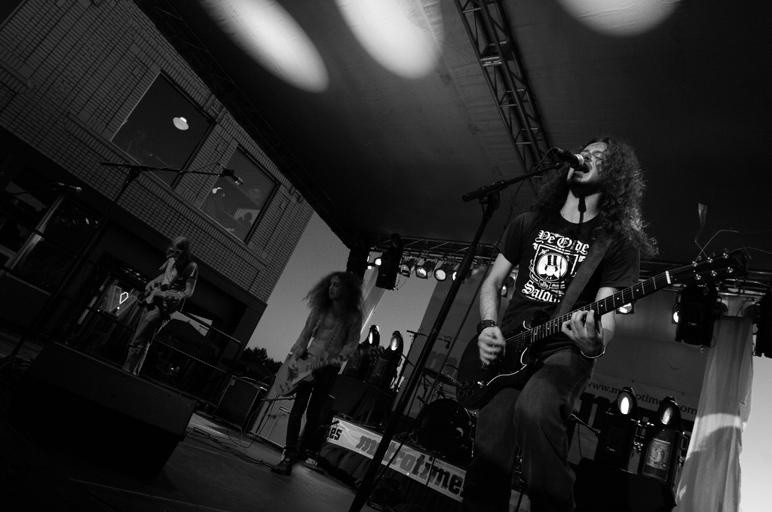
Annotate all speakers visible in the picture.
[7,337,199,479]
[217,374,268,430]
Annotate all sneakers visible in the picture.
[299,437,318,466]
[271,457,294,475]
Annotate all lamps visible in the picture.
[401,251,479,283]
[672,285,729,348]
[614,387,683,428]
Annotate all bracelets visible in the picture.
[580,344,607,359]
[477,319,496,334]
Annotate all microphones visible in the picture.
[560,148,584,170]
[58,182,83,193]
[220,163,245,188]
[444,341,451,349]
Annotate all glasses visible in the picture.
[170,248,183,255]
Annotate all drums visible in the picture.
[416,399,475,457]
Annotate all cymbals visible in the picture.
[426,368,460,387]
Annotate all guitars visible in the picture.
[457,247,748,410]
[273,350,345,398]
[143,287,181,305]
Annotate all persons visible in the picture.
[122,238,199,376]
[271,270,364,476]
[461,138,650,511]
